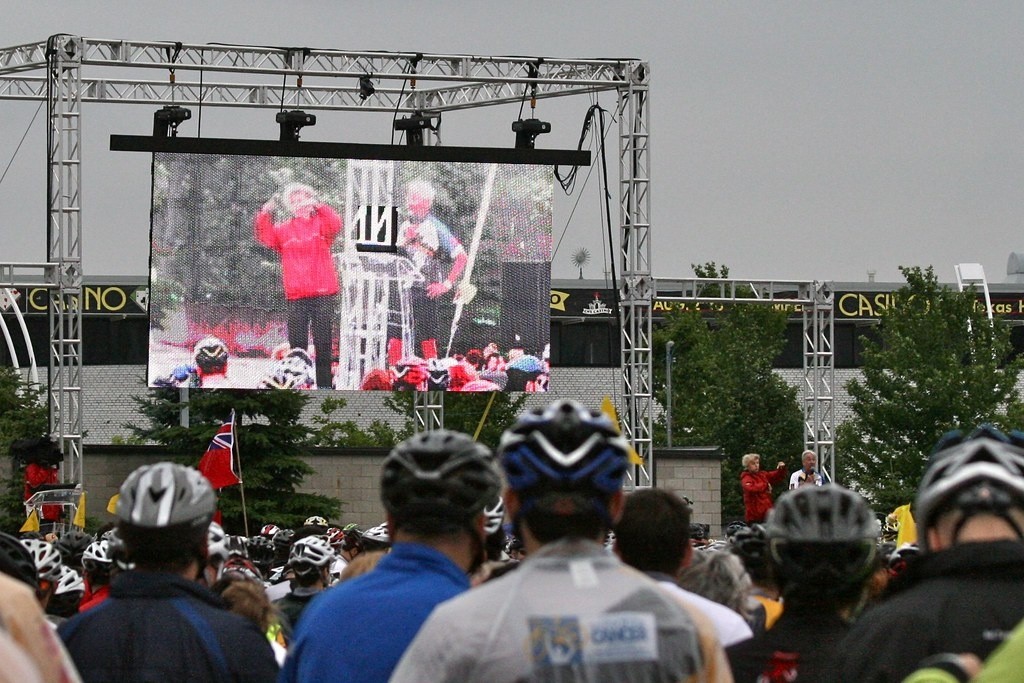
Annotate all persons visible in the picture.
[740,453,786,524]
[826,435,1024,682]
[0,435,968,682]
[274,429,505,683]
[788,449,823,491]
[194,177,548,389]
[57,462,282,682]
[383,177,468,366]
[251,180,344,394]
[391,401,734,682]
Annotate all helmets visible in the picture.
[764,486,880,547]
[193,337,230,365]
[261,347,550,392]
[0,484,921,596]
[911,427,1024,524]
[380,429,500,520]
[499,399,628,493]
[115,461,215,529]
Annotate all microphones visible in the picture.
[810,467,814,474]
[408,211,413,223]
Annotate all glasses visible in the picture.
[38,580,53,592]
[537,379,542,384]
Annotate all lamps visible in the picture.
[276,113,316,126]
[394,118,426,130]
[157,108,191,122]
[512,121,551,133]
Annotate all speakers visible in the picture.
[499,261,551,361]
